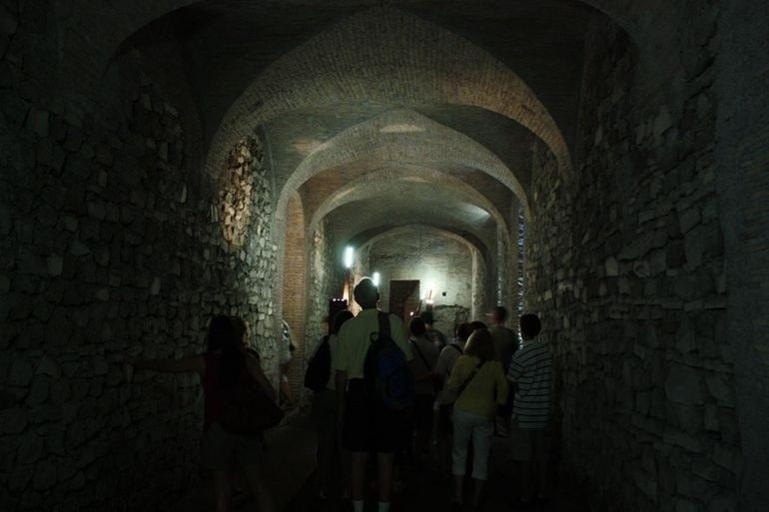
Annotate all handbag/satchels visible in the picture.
[303,335,332,393]
[219,384,285,433]
[458,461,502,497]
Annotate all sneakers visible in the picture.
[453,501,482,512]
[509,495,550,512]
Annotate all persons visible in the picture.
[281,317,299,404]
[201,312,283,511]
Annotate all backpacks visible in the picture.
[363,312,416,409]
